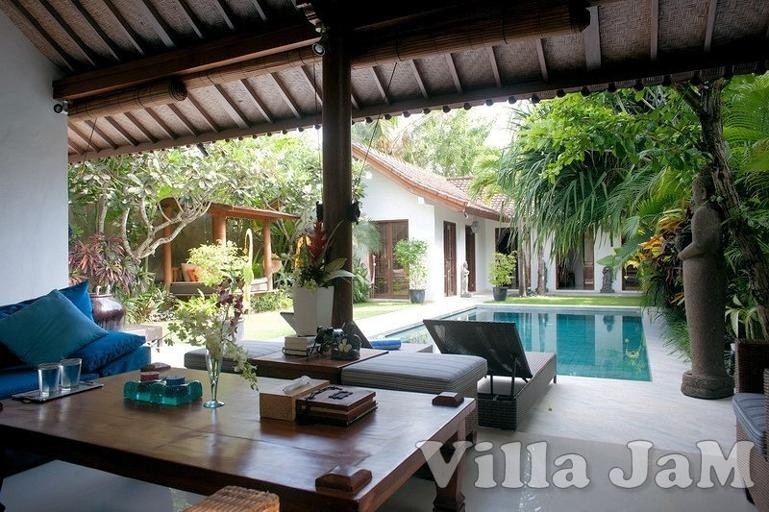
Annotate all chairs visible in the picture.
[422,318,560,429]
[0,284,150,395]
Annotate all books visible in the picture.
[296,384,379,427]
[281,335,317,357]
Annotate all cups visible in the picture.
[59,357,83,391]
[37,361,60,397]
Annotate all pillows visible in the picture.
[2,290,144,374]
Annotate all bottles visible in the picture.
[120,370,203,406]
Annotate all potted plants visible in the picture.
[486,250,518,301]
[394,237,428,302]
[271,217,362,336]
